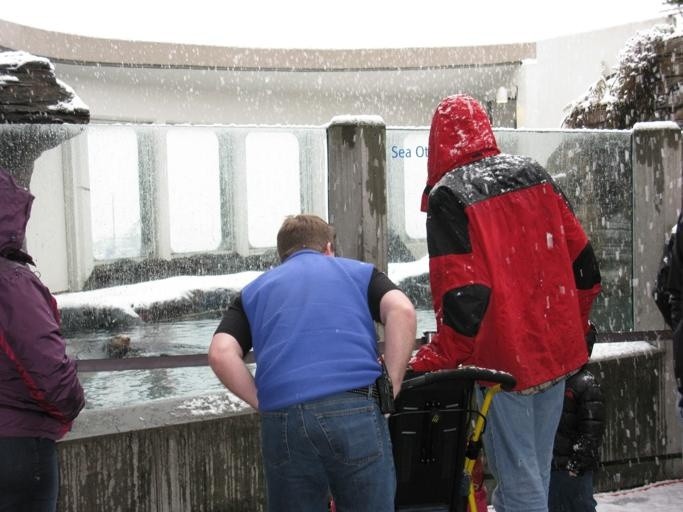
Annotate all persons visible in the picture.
[0,165,85,512]
[405,94,601,511]
[207,213,417,512]
[549,323,604,511]
[654,210,682,418]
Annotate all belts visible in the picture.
[345,383,379,401]
